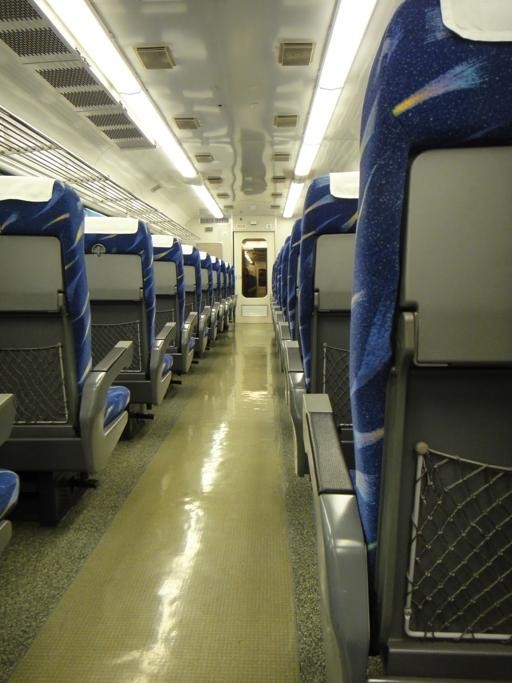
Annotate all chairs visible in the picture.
[2,175,238,555]
[265,0,511,683]
